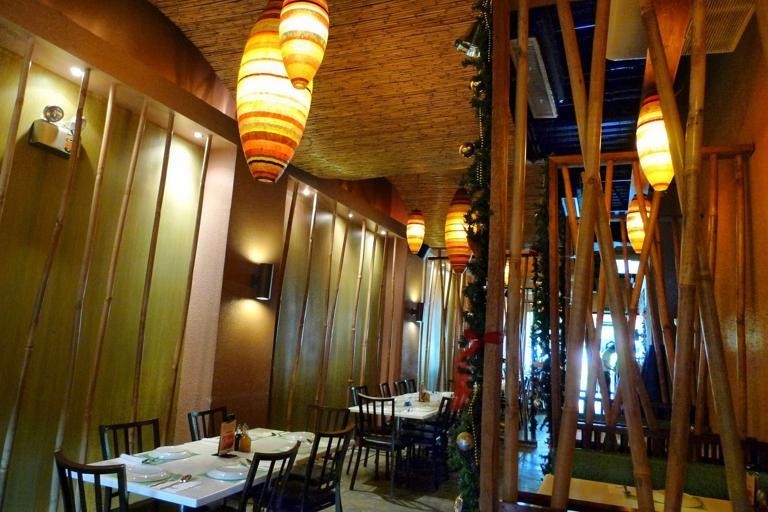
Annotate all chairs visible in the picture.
[346,377,466,502]
[250,422,356,511]
[54,448,129,512]
[187,406,227,442]
[306,404,350,436]
[184,439,302,511]
[98,418,161,512]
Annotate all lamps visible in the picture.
[410,302,424,323]
[626,193,652,254]
[234,0,314,185]
[406,207,426,255]
[278,0,331,90]
[250,264,274,301]
[635,89,675,192]
[444,187,474,274]
[27,105,86,160]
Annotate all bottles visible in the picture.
[236,423,253,454]
[32,105,86,154]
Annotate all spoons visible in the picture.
[151,475,194,492]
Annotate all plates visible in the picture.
[206,466,252,481]
[123,463,172,482]
[146,444,197,458]
[253,428,321,453]
[372,391,453,416]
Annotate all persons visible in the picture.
[599,344,616,399]
